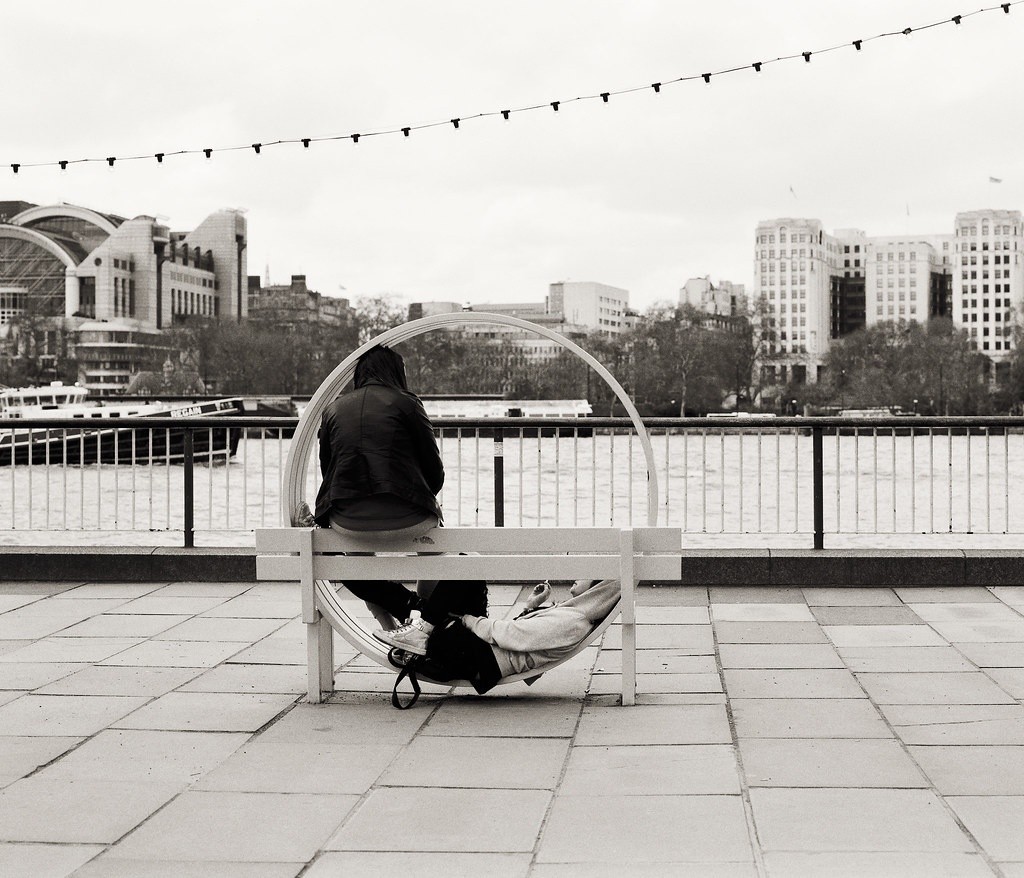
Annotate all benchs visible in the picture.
[254,311,682,705]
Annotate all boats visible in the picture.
[0,379,244,466]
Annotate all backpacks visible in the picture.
[393,616,489,710]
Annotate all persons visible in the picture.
[317,345,445,625]
[293,501,621,695]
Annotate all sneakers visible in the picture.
[294,501,322,555]
[372,618,434,656]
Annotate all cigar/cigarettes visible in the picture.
[543,580,549,583]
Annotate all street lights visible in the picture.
[913,399,918,415]
[791,399,798,415]
[841,367,845,409]
[671,400,676,417]
